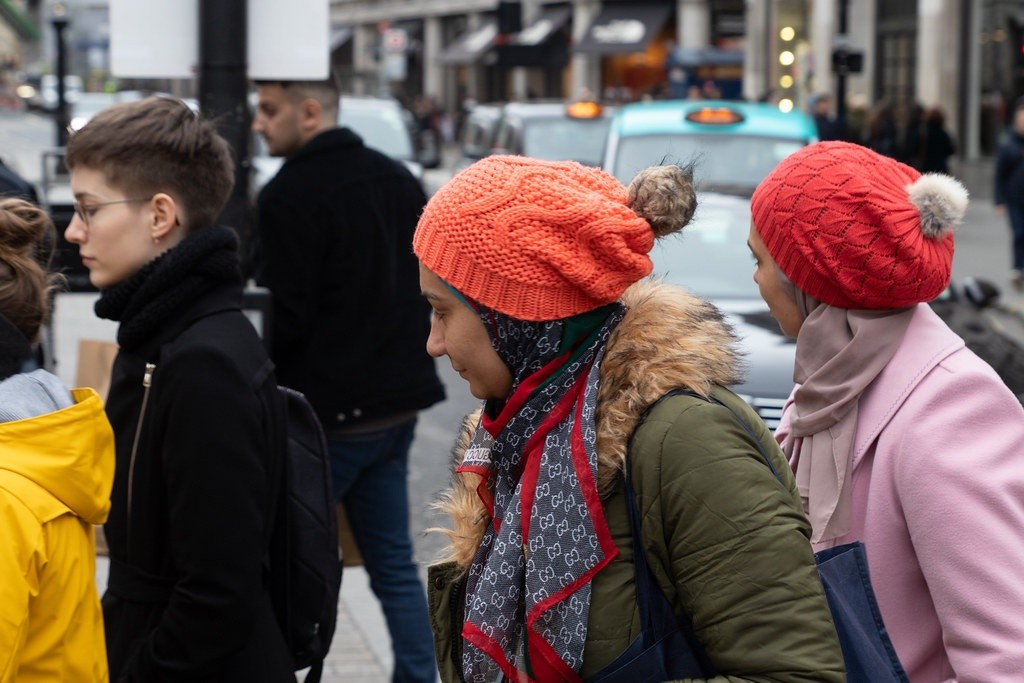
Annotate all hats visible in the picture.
[751,140,970,312]
[413,155,698,324]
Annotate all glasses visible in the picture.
[74,197,182,228]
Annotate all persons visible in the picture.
[412,155,845,683]
[66,93,342,683]
[247,70,448,683]
[993,106,1024,284]
[389,80,445,169]
[746,141,1024,683]
[812,97,957,176]
[0,160,115,683]
[604,80,720,105]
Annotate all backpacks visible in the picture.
[275,384,343,683]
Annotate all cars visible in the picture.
[14,71,821,441]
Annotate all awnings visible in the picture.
[441,0,673,66]
[330,26,352,57]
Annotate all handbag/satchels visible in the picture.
[588,389,911,683]
